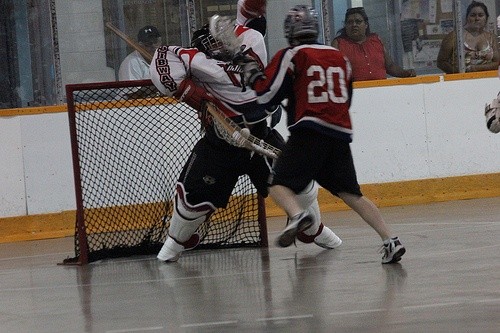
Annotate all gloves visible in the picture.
[235,54,263,89]
[173,79,214,111]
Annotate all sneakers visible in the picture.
[381,237,405,263]
[297,224,342,249]
[278,211,314,248]
[156,233,199,263]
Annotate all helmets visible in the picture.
[138,26,160,42]
[190,23,234,59]
[283,4,320,37]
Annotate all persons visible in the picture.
[118,26,164,81]
[437,1,499,75]
[149,0,342,264]
[331,6,418,81]
[254,4,406,266]
[484,91,500,134]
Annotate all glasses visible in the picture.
[346,7,364,13]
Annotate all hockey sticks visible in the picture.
[106,21,282,159]
